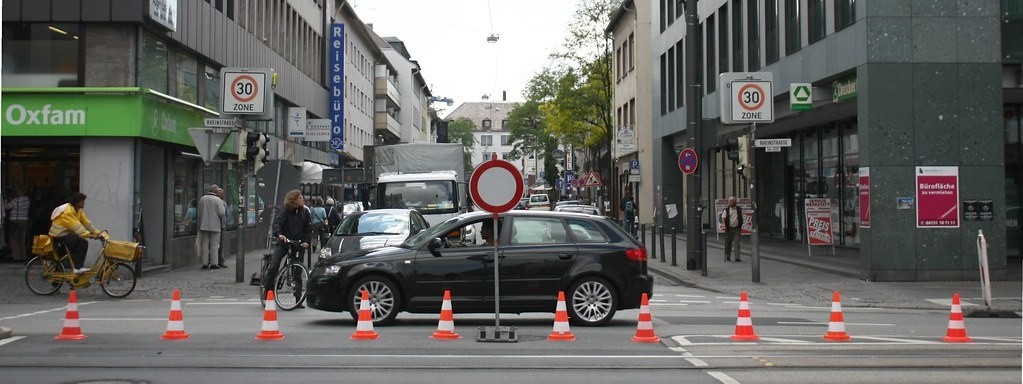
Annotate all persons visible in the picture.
[4,184,30,263]
[721,196,744,262]
[513,192,585,211]
[184,199,197,234]
[620,190,639,240]
[261,189,312,309]
[48,193,107,294]
[308,195,341,253]
[448,219,503,247]
[197,184,228,270]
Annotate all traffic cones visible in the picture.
[728,291,761,342]
[53,291,88,341]
[428,290,464,339]
[822,291,851,341]
[940,292,973,342]
[254,289,287,340]
[630,292,662,344]
[159,289,190,340]
[348,289,381,339]
[546,290,575,341]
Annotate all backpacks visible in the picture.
[624,199,634,213]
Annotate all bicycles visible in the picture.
[258,235,309,311]
[23,229,148,299]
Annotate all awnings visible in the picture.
[294,161,334,185]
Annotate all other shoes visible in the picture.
[312,246,316,252]
[726,256,730,261]
[210,265,220,269]
[73,269,90,273]
[201,264,208,270]
[735,258,742,262]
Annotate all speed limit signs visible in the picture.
[219,68,274,121]
[729,80,775,123]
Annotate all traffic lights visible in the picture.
[238,130,261,162]
[252,128,270,175]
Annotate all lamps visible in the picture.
[487,33,500,43]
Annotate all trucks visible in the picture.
[368,141,473,246]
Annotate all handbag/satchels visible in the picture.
[322,224,330,233]
[195,230,203,256]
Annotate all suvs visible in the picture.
[303,209,655,328]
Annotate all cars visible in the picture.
[246,193,265,213]
[317,206,431,261]
[514,192,607,243]
[465,211,477,245]
[171,186,198,224]
